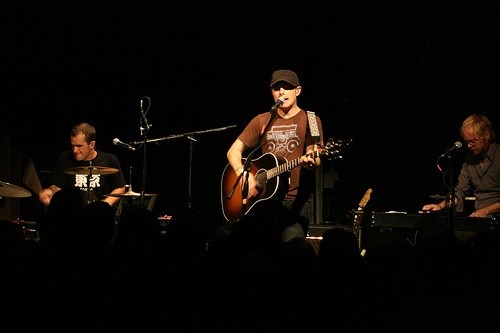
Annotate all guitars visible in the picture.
[220,138,352,223]
[353,188,373,258]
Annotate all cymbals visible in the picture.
[12,220,37,226]
[63,166,119,175]
[21,228,36,232]
[102,192,162,198]
[0,181,32,198]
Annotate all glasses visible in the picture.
[463,137,486,146]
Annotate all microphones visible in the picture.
[441,141,462,158]
[140,98,144,121]
[113,138,136,151]
[271,96,285,111]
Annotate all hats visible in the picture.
[269,69,299,87]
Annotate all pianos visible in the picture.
[371,211,495,256]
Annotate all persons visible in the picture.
[422,114,500,237]
[39,123,125,220]
[226,69,323,247]
